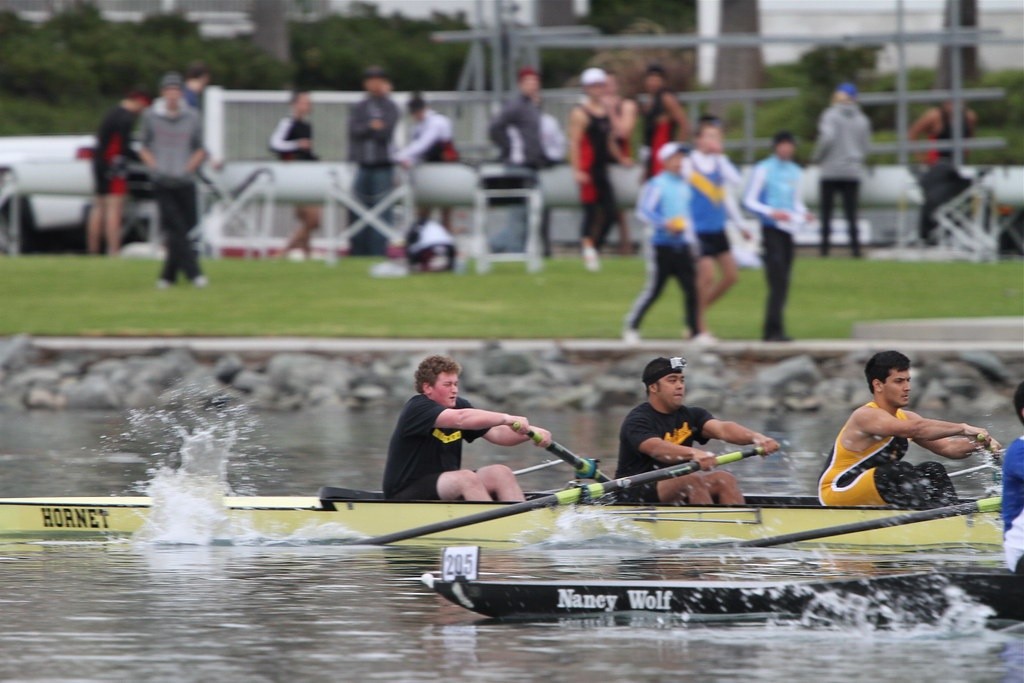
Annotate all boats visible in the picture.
[0,495,1003,553]
[421,545,1024,629]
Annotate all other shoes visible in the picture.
[689,334,720,347]
[624,325,641,347]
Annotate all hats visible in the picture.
[658,141,690,160]
[581,68,607,85]
[158,73,182,89]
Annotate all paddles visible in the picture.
[510,419,613,485]
[362,442,782,546]
[745,496,1004,548]
[977,433,1004,467]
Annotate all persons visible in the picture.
[641,64,689,180]
[184,58,223,173]
[266,90,321,257]
[345,64,398,254]
[623,142,718,342]
[817,349,1001,510]
[906,97,978,243]
[807,82,874,257]
[486,67,551,255]
[383,352,552,501]
[739,130,816,343]
[604,68,637,255]
[388,92,459,231]
[567,66,633,272]
[613,356,780,505]
[129,69,211,289]
[684,114,752,336]
[86,84,150,255]
[999,390,1024,574]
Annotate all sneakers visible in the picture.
[154,278,168,289]
[188,274,207,288]
[581,247,601,271]
